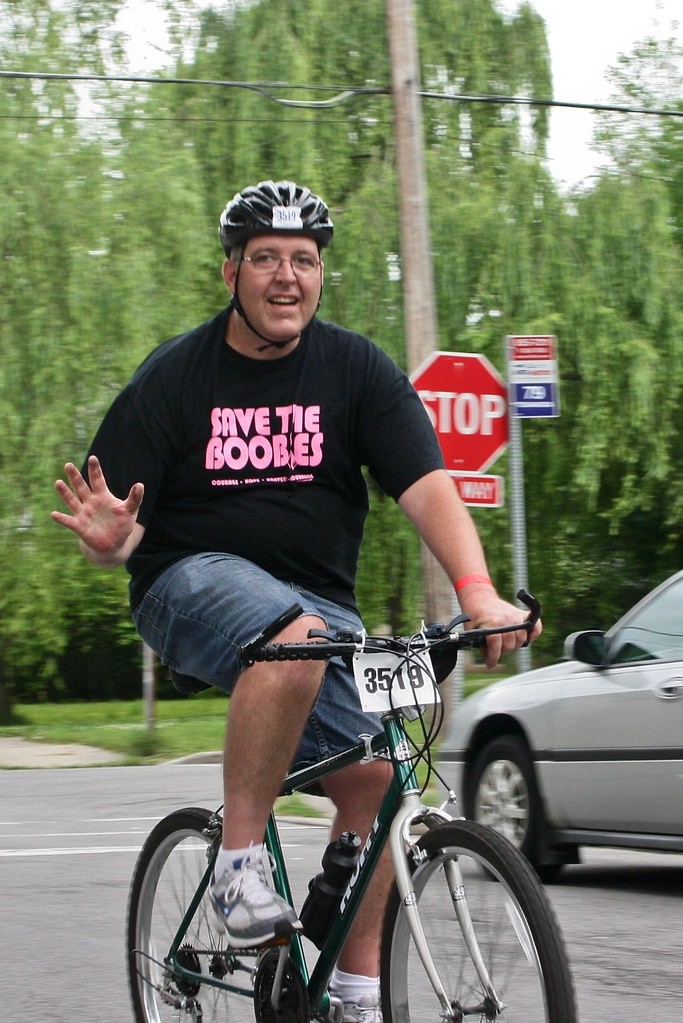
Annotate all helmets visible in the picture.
[221,180,334,259]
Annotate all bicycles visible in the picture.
[126,587,580,1023]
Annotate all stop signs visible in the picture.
[406,350,513,477]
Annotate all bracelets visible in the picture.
[453,573,493,595]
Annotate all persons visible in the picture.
[51,180,543,1022]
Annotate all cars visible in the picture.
[432,564,683,880]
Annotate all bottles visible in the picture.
[297,830,363,949]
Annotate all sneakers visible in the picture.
[208,866,303,948]
[321,991,387,1023]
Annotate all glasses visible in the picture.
[232,253,321,277]
